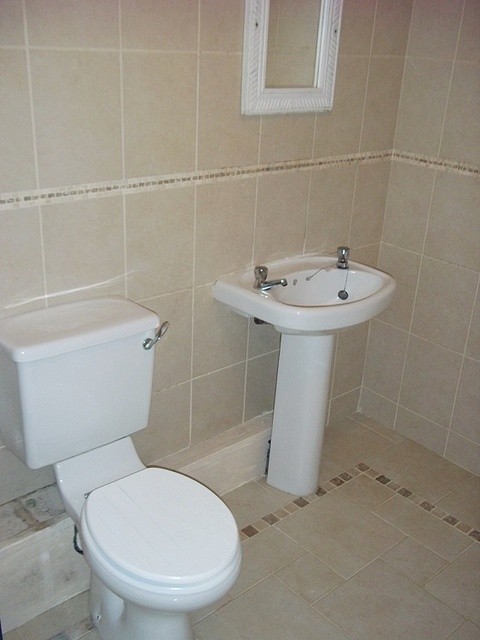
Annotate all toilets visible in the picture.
[0,293,244,639]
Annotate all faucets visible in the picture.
[253,263,288,290]
[337,245,350,271]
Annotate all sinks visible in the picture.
[274,269,385,306]
